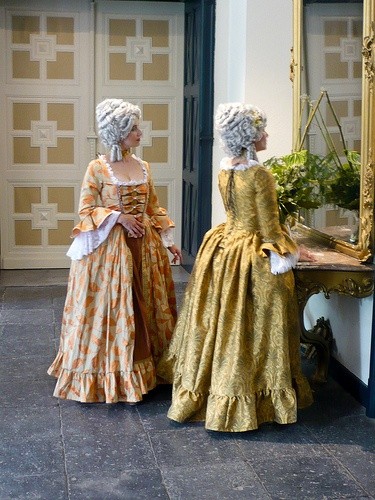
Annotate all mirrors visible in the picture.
[290,0,375,262]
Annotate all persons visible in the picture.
[156,103,317,433]
[46,98,184,406]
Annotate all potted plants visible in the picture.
[263,149,322,238]
[315,149,363,246]
